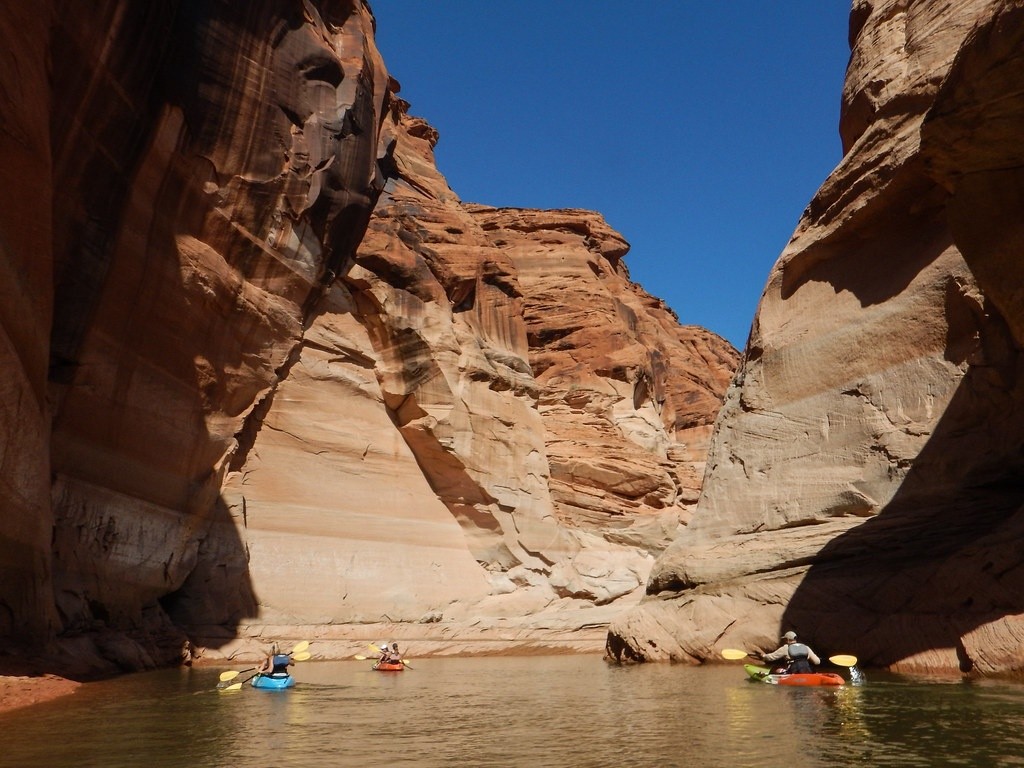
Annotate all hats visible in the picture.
[781,631,796,639]
[381,645,387,649]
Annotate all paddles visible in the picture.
[354,654,410,664]
[219,651,311,682]
[367,644,414,670]
[225,640,309,690]
[720,647,858,668]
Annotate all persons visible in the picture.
[758,632,820,674]
[182,641,192,666]
[255,642,295,678]
[378,643,404,664]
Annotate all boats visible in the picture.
[373,658,407,671]
[743,663,853,686]
[251,672,293,689]
